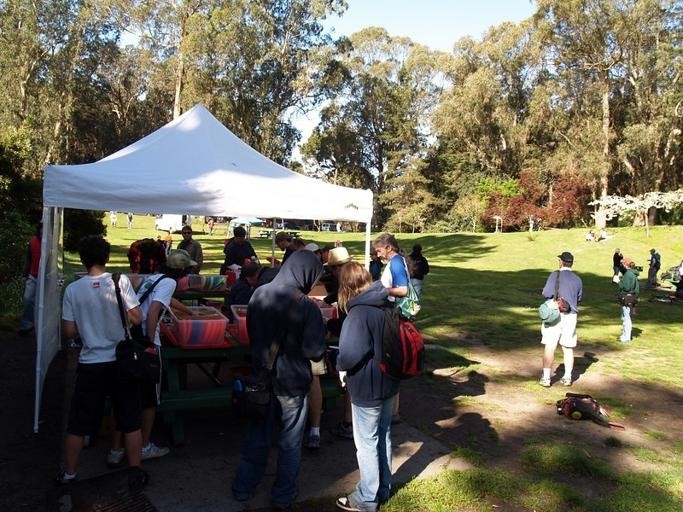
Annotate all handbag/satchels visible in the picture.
[395,284,421,320]
[556,392,609,427]
[617,291,637,306]
[539,299,560,323]
[116,338,160,384]
[232,385,283,427]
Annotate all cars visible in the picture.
[277,221,302,230]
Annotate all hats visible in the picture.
[241,263,261,275]
[558,253,573,262]
[322,247,355,266]
[275,231,292,241]
[620,258,632,266]
[649,248,655,253]
[304,243,321,252]
[165,249,199,268]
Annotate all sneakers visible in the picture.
[540,377,551,386]
[235,484,253,500]
[560,376,571,386]
[51,471,76,493]
[270,490,298,509]
[336,496,361,511]
[309,435,320,447]
[392,416,401,426]
[330,424,353,439]
[141,442,169,460]
[107,448,125,467]
[128,467,150,493]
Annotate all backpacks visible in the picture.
[370,305,425,377]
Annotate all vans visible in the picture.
[321,222,341,231]
[154,212,191,233]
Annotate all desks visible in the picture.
[158,336,338,448]
[173,289,225,306]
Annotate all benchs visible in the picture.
[157,374,341,447]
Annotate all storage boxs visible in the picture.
[231,305,249,344]
[169,306,229,345]
[176,274,190,292]
[189,274,228,293]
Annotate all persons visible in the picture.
[19,220,45,331]
[332,261,407,512]
[611,248,623,283]
[538,252,584,387]
[227,248,329,508]
[585,227,609,243]
[616,258,640,342]
[674,260,683,302]
[105,237,207,467]
[110,211,429,447]
[53,236,153,490]
[647,248,661,286]
